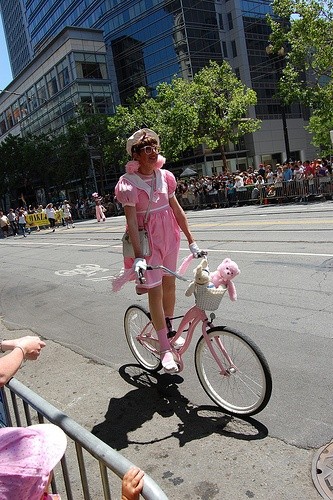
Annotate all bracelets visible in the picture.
[14,345,25,360]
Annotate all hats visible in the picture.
[92,193,98,197]
[46,203,53,208]
[125,128,160,157]
[64,199,69,202]
[234,176,242,180]
[0,423,68,499]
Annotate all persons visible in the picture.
[112,129,207,374]
[0,191,125,239]
[0,335,46,428]
[0,424,145,500]
[175,155,333,211]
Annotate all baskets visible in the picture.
[196,283,229,311]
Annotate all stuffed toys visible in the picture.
[186,258,241,301]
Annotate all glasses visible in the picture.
[140,146,160,154]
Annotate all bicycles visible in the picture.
[123,249,272,417]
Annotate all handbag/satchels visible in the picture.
[121,226,151,257]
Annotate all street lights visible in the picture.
[266,43,292,165]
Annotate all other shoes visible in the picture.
[169,329,185,348]
[158,348,178,374]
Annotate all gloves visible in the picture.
[132,257,147,279]
[188,241,201,259]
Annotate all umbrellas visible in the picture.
[179,168,197,179]
